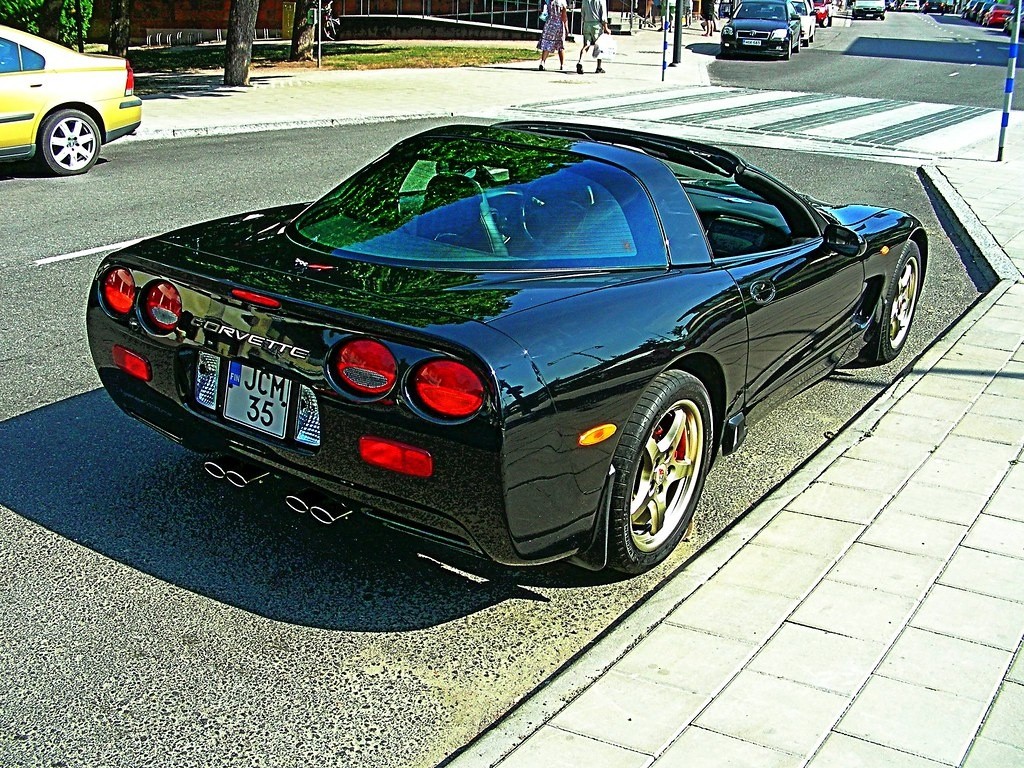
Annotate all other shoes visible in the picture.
[539,64,546,71]
[595,67,606,73]
[713,29,720,33]
[669,30,672,32]
[683,25,688,27]
[703,33,712,37]
[657,28,663,31]
[576,63,584,74]
[560,66,563,70]
[700,24,706,31]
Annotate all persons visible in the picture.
[637,0,692,33]
[699,0,721,37]
[536,0,568,72]
[576,0,612,75]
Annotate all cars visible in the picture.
[852,0,888,21]
[720,0,803,61]
[946,0,1024,34]
[0,26,144,176]
[901,0,921,13]
[791,0,834,47]
[921,0,946,16]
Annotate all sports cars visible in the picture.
[86,117,930,574]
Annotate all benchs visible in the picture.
[757,12,772,17]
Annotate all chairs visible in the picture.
[774,7,784,18]
[575,200,636,256]
[417,175,509,255]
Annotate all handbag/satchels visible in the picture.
[592,30,618,60]
[538,4,549,23]
